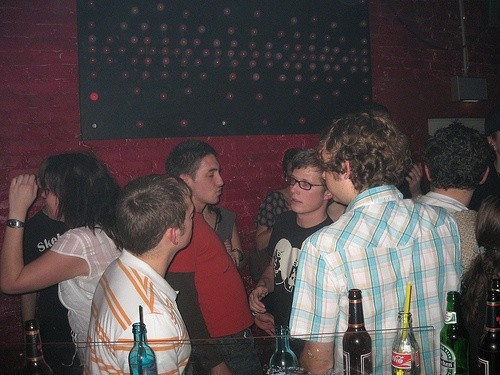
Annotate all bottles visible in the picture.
[477,278,500,375]
[19,320,53,375]
[269,325,299,375]
[342,289,373,375]
[390,311,421,375]
[128,322,158,375]
[439,291,467,375]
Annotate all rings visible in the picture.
[252,311,257,317]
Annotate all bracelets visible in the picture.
[232,248,243,261]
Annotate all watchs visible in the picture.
[6,219,25,228]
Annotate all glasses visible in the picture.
[284,175,327,191]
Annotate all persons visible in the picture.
[0,152,123,367]
[249,101,500,375]
[83,174,194,375]
[202,204,244,267]
[165,141,261,375]
[289,112,462,375]
